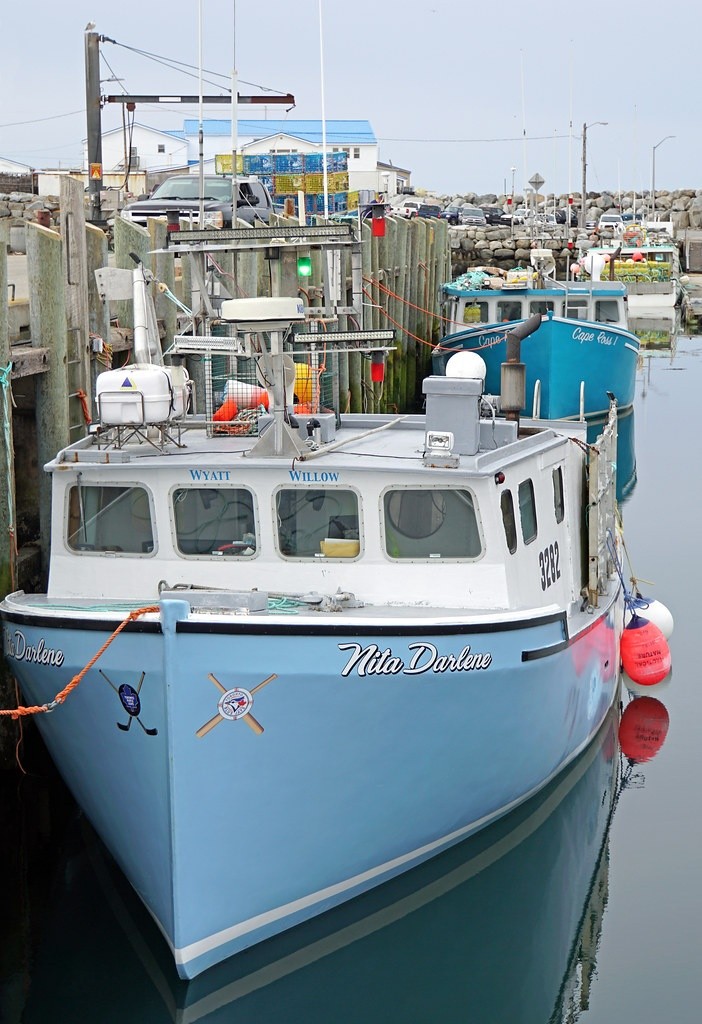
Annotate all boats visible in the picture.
[0,677,630,1024]
[585,408,638,506]
[0,1,625,983]
[431,46,682,423]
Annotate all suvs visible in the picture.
[121,173,277,259]
[417,204,596,229]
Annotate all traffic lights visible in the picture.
[298,257,312,278]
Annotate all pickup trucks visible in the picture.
[390,201,425,221]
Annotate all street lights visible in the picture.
[581,122,608,226]
[652,136,675,220]
[511,166,516,239]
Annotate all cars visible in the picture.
[622,213,646,220]
[598,215,622,231]
